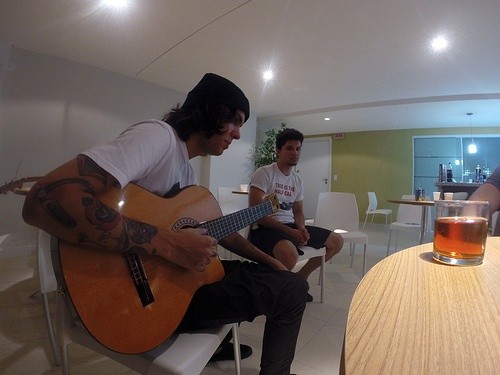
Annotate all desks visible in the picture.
[434,182,484,201]
[387,198,435,244]
[339,235,500,375]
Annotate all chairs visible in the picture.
[311,191,369,278]
[386,194,431,258]
[361,192,393,235]
[37,187,326,375]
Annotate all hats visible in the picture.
[182,72,250,124]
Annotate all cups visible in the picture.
[432,200,489,266]
[433,192,441,200]
[240,184,248,192]
[444,193,453,200]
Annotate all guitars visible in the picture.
[50,181,280,355]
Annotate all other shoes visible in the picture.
[208,342,252,362]
[305,293,313,302]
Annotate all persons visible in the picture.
[247,129,344,302]
[22,73,309,375]
[460,165,500,218]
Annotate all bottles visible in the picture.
[476,165,482,180]
[415,185,425,201]
[447,163,453,182]
